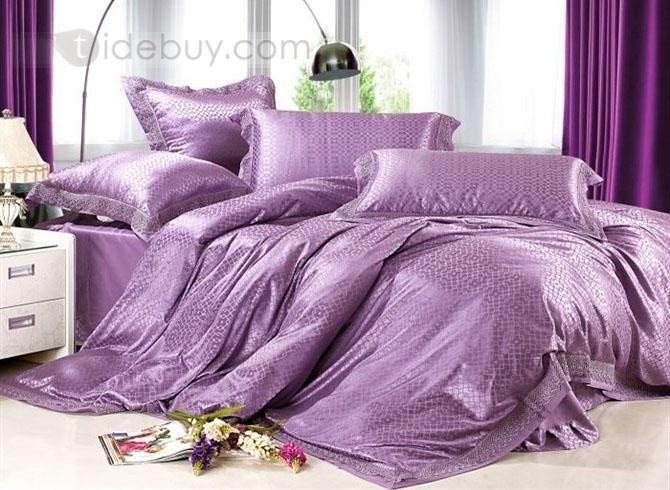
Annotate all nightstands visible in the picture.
[0,226,78,367]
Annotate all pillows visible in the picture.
[336,152,610,242]
[24,149,254,245]
[230,107,460,194]
[120,76,276,174]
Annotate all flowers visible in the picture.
[166,410,307,477]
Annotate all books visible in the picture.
[98,420,282,466]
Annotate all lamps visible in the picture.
[0,109,51,250]
[81,0,362,161]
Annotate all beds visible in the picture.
[0,201,670,490]
